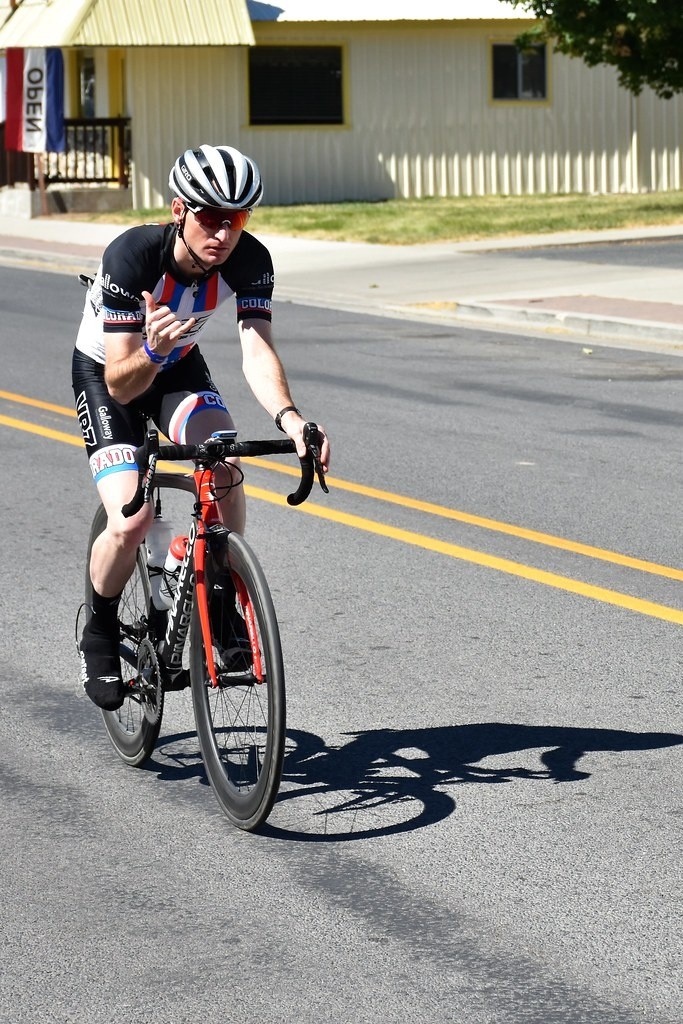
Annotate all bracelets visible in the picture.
[145,342,167,363]
[275,407,302,433]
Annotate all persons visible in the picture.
[71,144,330,710]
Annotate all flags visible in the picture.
[5,47,68,154]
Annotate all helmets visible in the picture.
[169,143,263,210]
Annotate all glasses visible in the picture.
[184,202,252,231]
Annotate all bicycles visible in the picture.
[76,420,336,836]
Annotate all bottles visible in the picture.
[144,515,172,610]
[158,536,189,607]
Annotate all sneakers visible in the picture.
[208,606,262,671]
[80,620,125,711]
